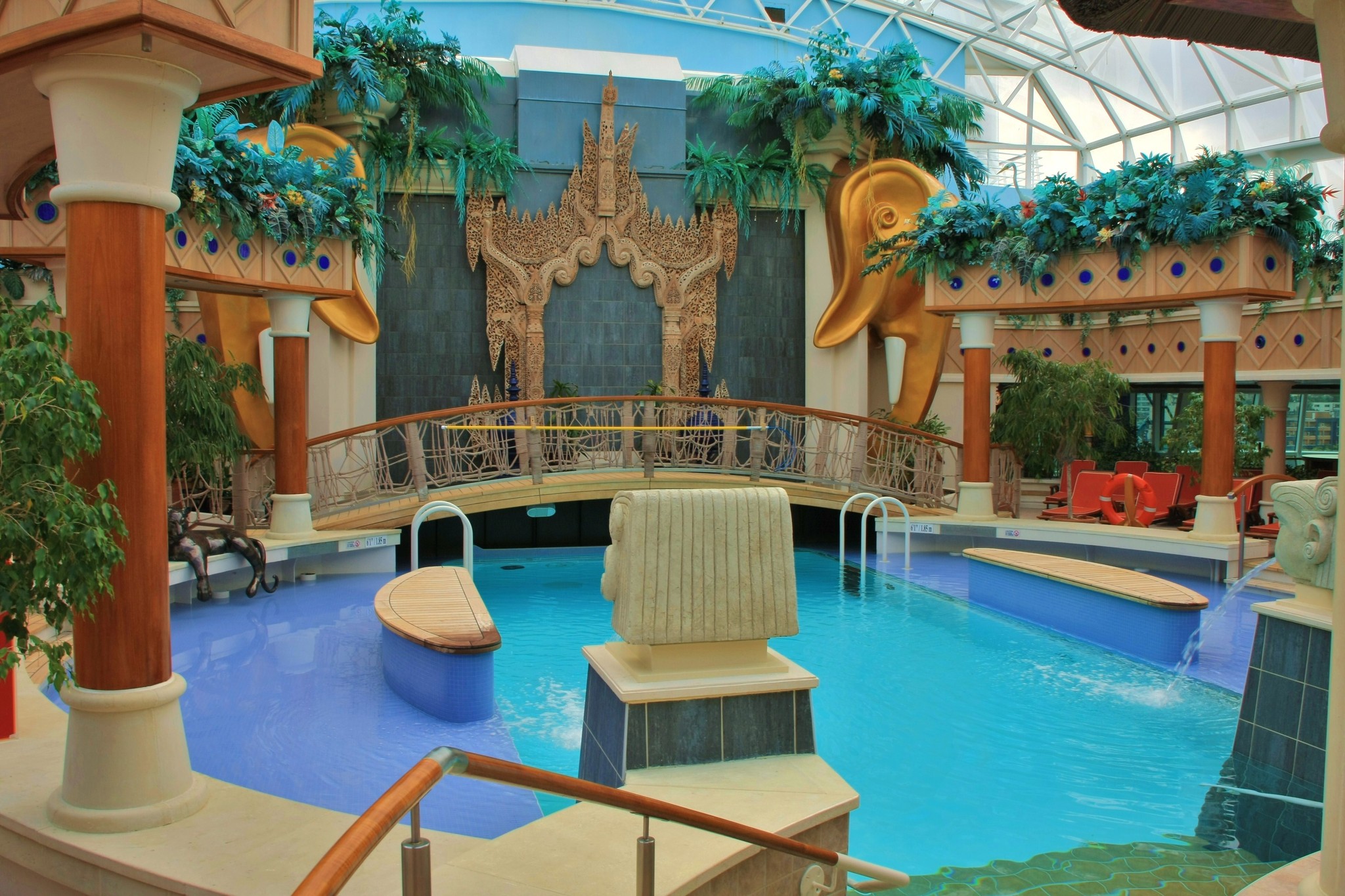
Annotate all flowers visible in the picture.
[684,29,1345,347]
[26,101,387,287]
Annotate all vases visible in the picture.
[0,180,359,300]
[926,227,1297,313]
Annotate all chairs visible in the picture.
[1037,460,1298,539]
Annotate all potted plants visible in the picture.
[242,0,539,228]
[0,295,131,741]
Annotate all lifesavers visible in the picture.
[1099,474,1156,527]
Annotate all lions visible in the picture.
[168,505,278,600]
